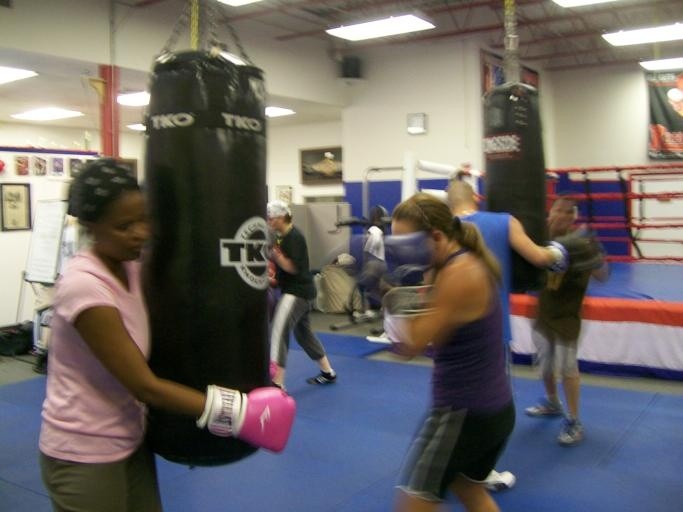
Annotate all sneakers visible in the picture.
[306,368,337,385]
[483,468,516,493]
[558,413,585,446]
[524,395,564,416]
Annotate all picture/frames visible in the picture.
[297,143,342,187]
[0,182,32,233]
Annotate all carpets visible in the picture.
[0,331,680,512]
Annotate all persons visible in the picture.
[264,201,338,390]
[446,179,569,494]
[525,196,608,447]
[38,158,296,512]
[381,192,515,512]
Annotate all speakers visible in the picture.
[343,56,361,78]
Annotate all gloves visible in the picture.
[195,384,297,452]
[384,231,433,314]
